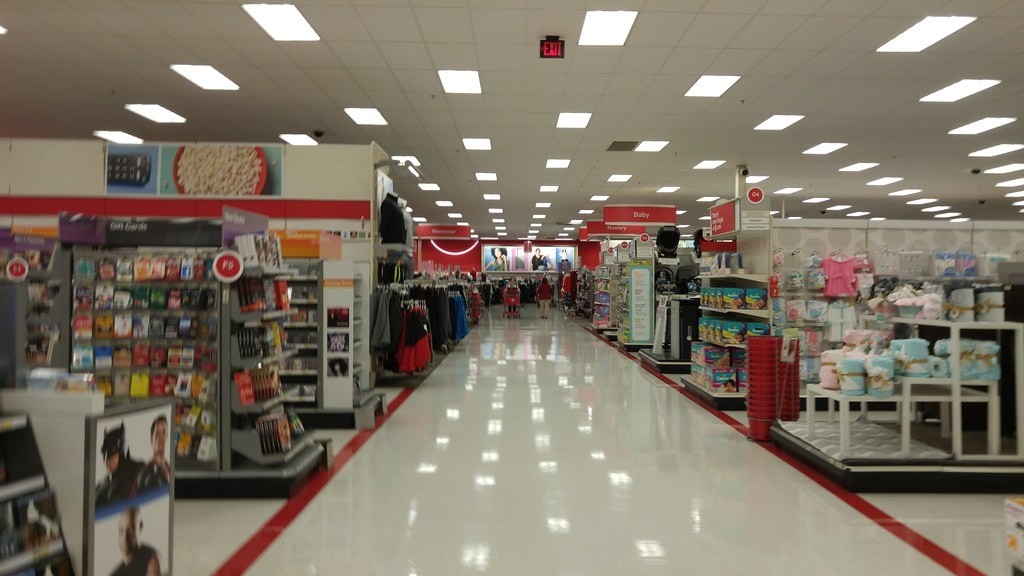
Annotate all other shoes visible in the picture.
[544,317,547,319]
[540,315,544,318]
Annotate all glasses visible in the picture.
[102,442,122,461]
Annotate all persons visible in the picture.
[109,508,161,576]
[379,191,413,248]
[559,248,570,270]
[95,420,147,510]
[505,276,521,318]
[131,415,170,498]
[559,270,577,300]
[485,248,509,271]
[470,268,476,281]
[537,278,553,319]
[532,248,556,271]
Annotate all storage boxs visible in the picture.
[691,252,771,394]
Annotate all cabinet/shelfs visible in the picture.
[221,204,333,499]
[557,247,701,374]
[273,229,387,430]
[768,277,1024,492]
[680,219,774,411]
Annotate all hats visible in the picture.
[101,421,124,462]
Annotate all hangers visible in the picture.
[377,277,479,312]
